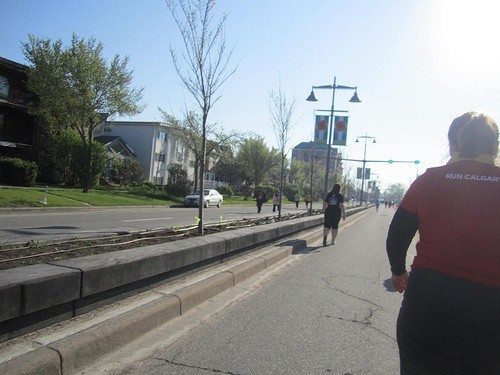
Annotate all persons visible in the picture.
[376,199,391,213]
[386,112,500,375]
[304,192,310,208]
[253,187,266,214]
[323,184,346,246]
[294,190,301,208]
[272,189,279,213]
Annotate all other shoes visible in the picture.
[332,242,336,244]
[323,238,328,246]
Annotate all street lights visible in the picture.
[306,77,362,213]
[355,132,377,207]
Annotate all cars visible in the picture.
[184,188,224,208]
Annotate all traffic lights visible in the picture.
[389,160,392,164]
[415,160,419,164]
